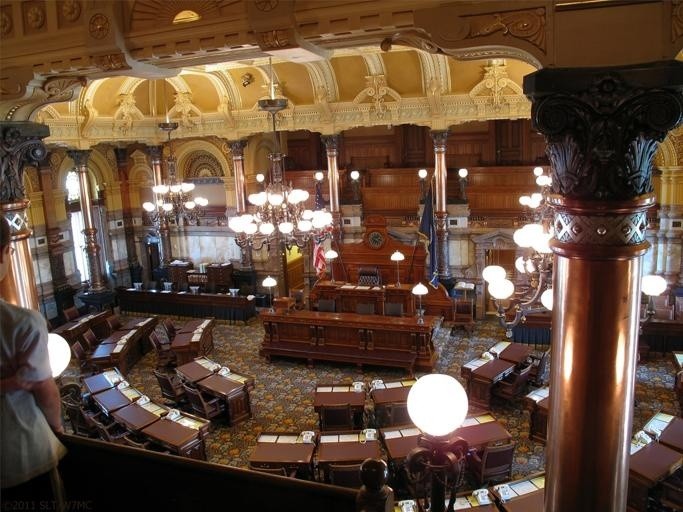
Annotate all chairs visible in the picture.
[471,441,516,488]
[248,465,297,479]
[656,480,682,511]
[329,463,363,488]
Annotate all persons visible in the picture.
[0,213,77,512]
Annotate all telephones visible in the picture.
[637,431,651,444]
[301,430,314,444]
[494,484,511,501]
[111,374,129,390]
[165,408,180,421]
[372,380,383,390]
[472,488,491,506]
[218,366,231,376]
[136,395,150,406]
[208,363,221,371]
[352,382,363,390]
[363,429,376,442]
[647,424,662,437]
[398,499,415,512]
[482,351,495,361]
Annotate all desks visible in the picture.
[114,282,259,326]
[638,296,683,362]
[47,306,255,461]
[379,412,512,491]
[529,384,550,447]
[259,266,474,379]
[445,470,546,511]
[461,339,551,412]
[643,407,683,453]
[313,377,416,432]
[628,430,683,512]
[317,429,381,482]
[247,429,319,481]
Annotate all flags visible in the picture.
[304,175,333,282]
[417,174,444,291]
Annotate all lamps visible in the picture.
[406,374,470,511]
[223,53,333,254]
[472,161,666,339]
[390,250,405,288]
[254,166,469,208]
[141,76,209,228]
[641,274,667,296]
